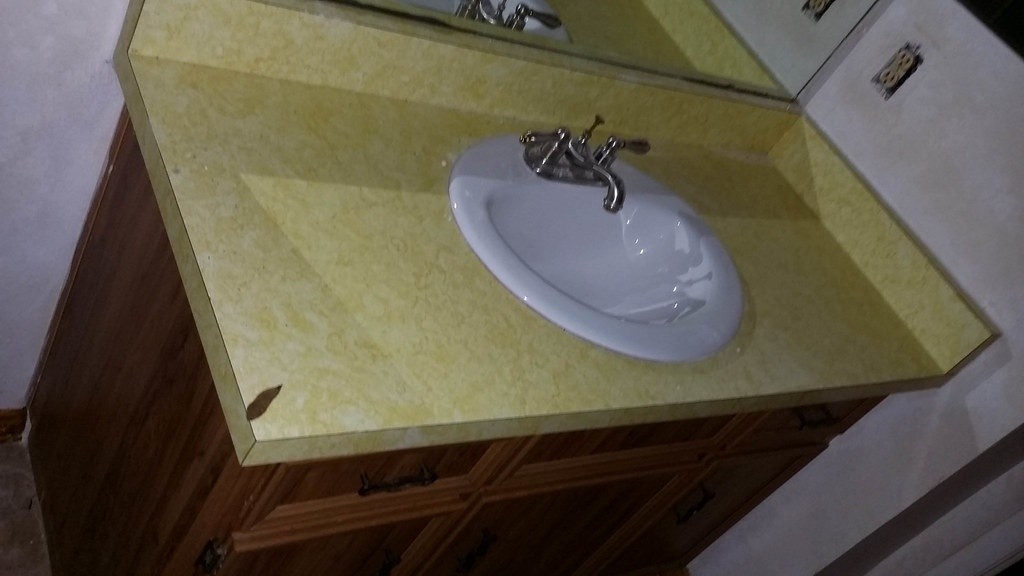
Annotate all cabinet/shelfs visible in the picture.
[19,101,886,576]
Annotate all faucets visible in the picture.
[453,0,562,32]
[519,114,652,214]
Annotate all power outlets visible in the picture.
[869,41,924,99]
[800,0,837,23]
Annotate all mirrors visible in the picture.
[334,0,882,102]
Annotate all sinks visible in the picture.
[403,0,568,44]
[447,132,745,362]
